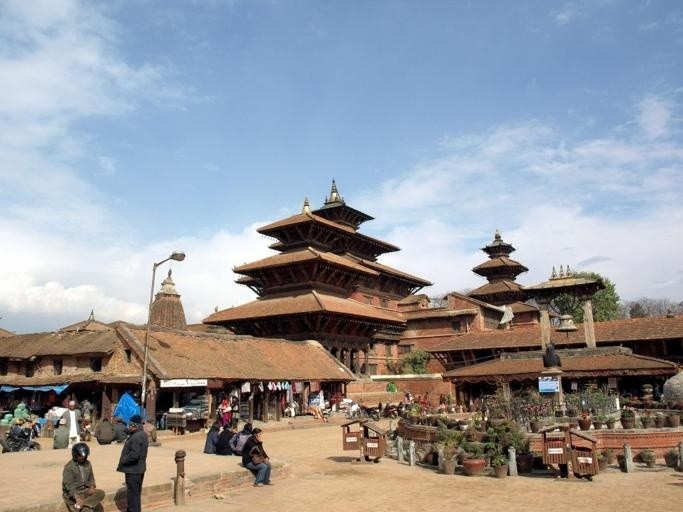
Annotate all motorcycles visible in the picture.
[360,402,404,421]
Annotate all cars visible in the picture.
[331,398,360,416]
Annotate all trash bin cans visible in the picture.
[362,422,386,462]
[569,427,599,479]
[340,418,368,450]
[537,423,569,478]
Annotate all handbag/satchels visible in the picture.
[248,445,265,465]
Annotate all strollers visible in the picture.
[0,425,39,452]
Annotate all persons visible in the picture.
[60,399,80,449]
[95,418,114,444]
[143,417,161,447]
[62,395,72,408]
[398,392,483,417]
[52,418,68,448]
[652,383,661,402]
[203,395,275,487]
[116,414,149,512]
[112,418,129,443]
[62,443,105,512]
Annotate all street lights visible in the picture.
[140,250,185,409]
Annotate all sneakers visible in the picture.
[263,480,272,484]
[254,482,263,487]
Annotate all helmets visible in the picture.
[71,443,89,462]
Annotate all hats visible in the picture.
[127,415,141,427]
[68,400,75,405]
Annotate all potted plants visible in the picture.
[407,383,682,479]
